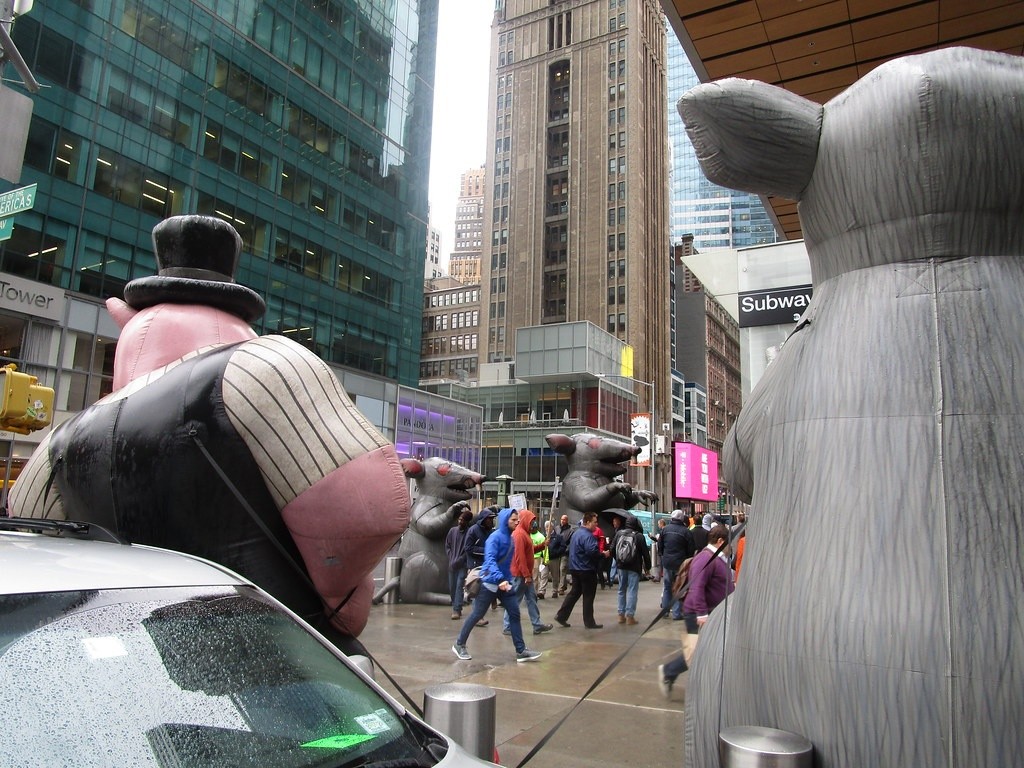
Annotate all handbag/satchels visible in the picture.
[463,566,484,599]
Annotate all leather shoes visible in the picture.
[554,615,570,627]
[588,624,603,628]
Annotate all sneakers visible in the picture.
[534,623,553,634]
[475,618,490,626]
[516,647,542,662]
[659,665,673,698]
[452,610,461,619]
[452,640,472,660]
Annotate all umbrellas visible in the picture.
[499,412,503,427]
[530,411,536,428]
[602,508,643,534]
[562,409,569,424]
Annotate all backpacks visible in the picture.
[616,529,640,565]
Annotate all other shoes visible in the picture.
[537,593,544,598]
[552,592,559,598]
[626,616,639,624]
[619,615,626,623]
[601,584,606,590]
[559,590,564,595]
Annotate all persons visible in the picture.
[610,517,651,626]
[605,518,624,587]
[502,509,553,635]
[691,514,746,582]
[658,525,734,697]
[537,521,566,599]
[464,510,496,627]
[453,509,542,663]
[445,511,472,619]
[556,515,573,595]
[530,521,549,594]
[554,512,610,628]
[648,510,695,620]
[594,521,605,589]
